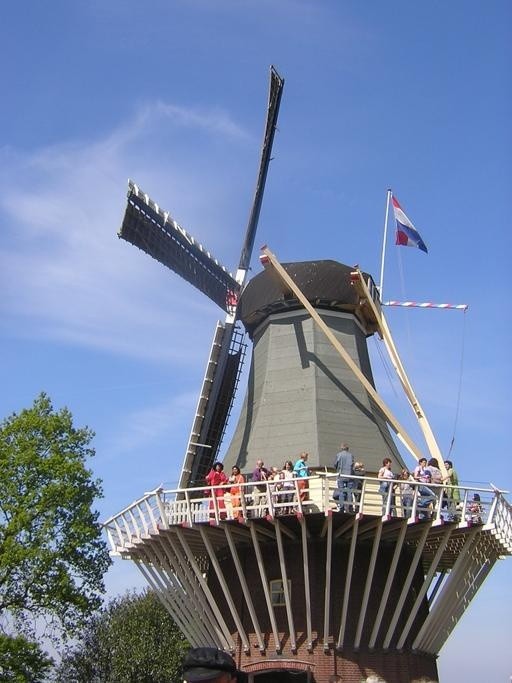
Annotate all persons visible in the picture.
[465,493,483,522]
[268,465,284,516]
[398,467,413,516]
[294,450,312,514]
[252,458,271,517]
[414,458,434,518]
[278,460,294,515]
[336,441,353,511]
[205,461,229,522]
[354,460,366,511]
[426,457,441,504]
[442,460,460,521]
[182,646,238,683]
[227,465,246,519]
[378,457,394,514]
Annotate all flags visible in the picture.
[391,191,428,253]
